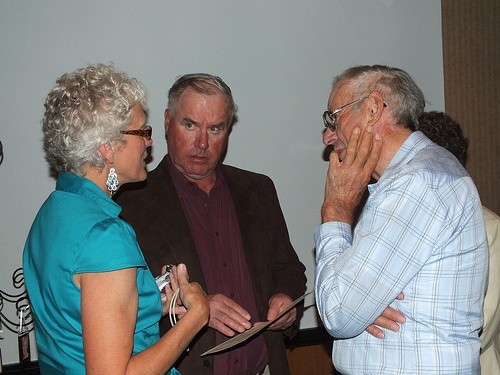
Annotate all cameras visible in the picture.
[155,264,173,293]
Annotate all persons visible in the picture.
[407,110,500,375]
[312,62,490,375]
[114,72,309,375]
[21,60,212,375]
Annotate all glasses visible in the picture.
[322,95,387,131]
[120,125,152,140]
